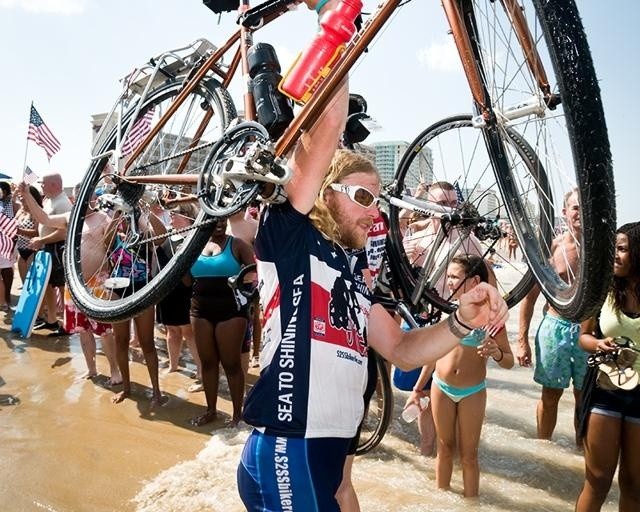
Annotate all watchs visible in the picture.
[447,313,468,339]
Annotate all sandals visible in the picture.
[607,338,638,363]
[593,357,639,391]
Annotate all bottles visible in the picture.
[401,396,431,423]
[282,0,363,99]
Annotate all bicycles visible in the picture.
[226,115,556,456]
[64,0,618,324]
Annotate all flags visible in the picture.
[453,180,464,203]
[27,106,60,164]
[117,104,154,157]
[23,167,39,184]
[1,214,18,262]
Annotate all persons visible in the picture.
[13,183,43,325]
[1,275,8,312]
[157,199,206,382]
[29,178,72,338]
[393,181,483,457]
[576,222,639,508]
[99,198,168,407]
[15,180,123,385]
[516,189,591,447]
[1,183,20,308]
[399,182,432,233]
[188,202,258,392]
[252,300,264,365]
[129,321,142,350]
[176,212,255,430]
[364,285,404,429]
[490,215,524,260]
[237,0,509,511]
[403,254,514,496]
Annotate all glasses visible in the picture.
[329,183,380,210]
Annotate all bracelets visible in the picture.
[313,0,325,9]
[412,387,421,392]
